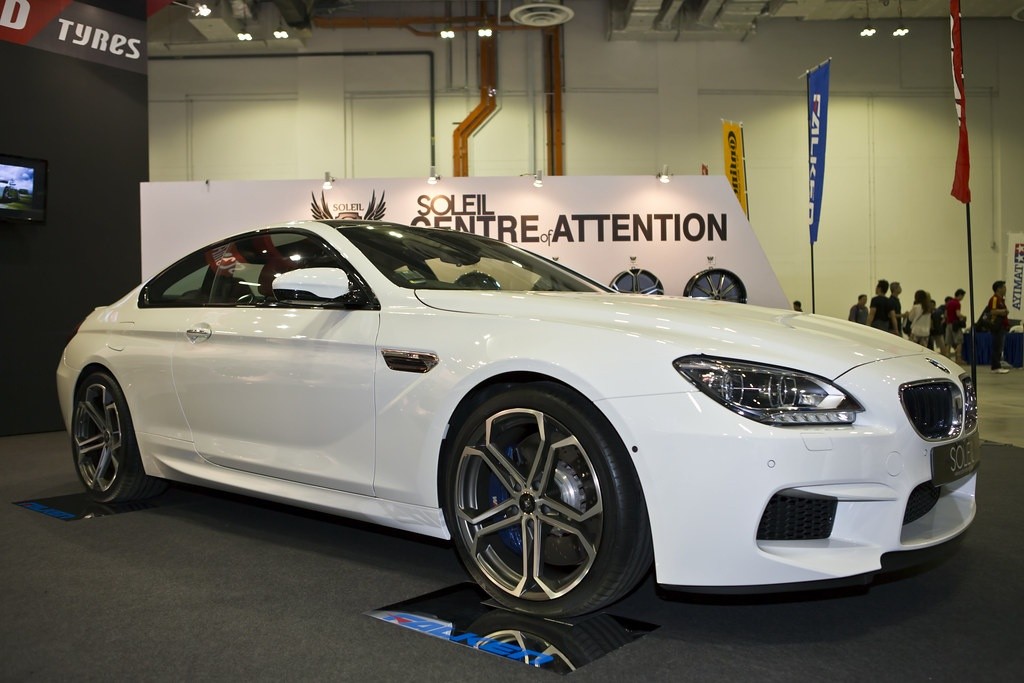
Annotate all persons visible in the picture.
[988,282,1011,373]
[848,295,868,325]
[866,280,908,338]
[945,289,966,363]
[905,290,952,356]
[793,301,802,312]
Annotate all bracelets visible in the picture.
[895,329,898,330]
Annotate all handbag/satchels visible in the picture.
[902,314,912,335]
[983,311,1003,332]
[953,319,967,332]
[976,306,991,333]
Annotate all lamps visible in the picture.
[655,164,670,184]
[323,172,335,189]
[172,1,212,16]
[534,169,543,187]
[427,174,440,185]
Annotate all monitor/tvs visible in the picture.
[0,158,46,221]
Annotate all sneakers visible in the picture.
[991,367,1009,374]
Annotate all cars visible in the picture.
[55,220,980,620]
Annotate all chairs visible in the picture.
[257,255,297,307]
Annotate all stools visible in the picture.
[960,330,1024,369]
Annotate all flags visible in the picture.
[719,119,748,218]
[948,1,971,204]
[808,62,831,244]
